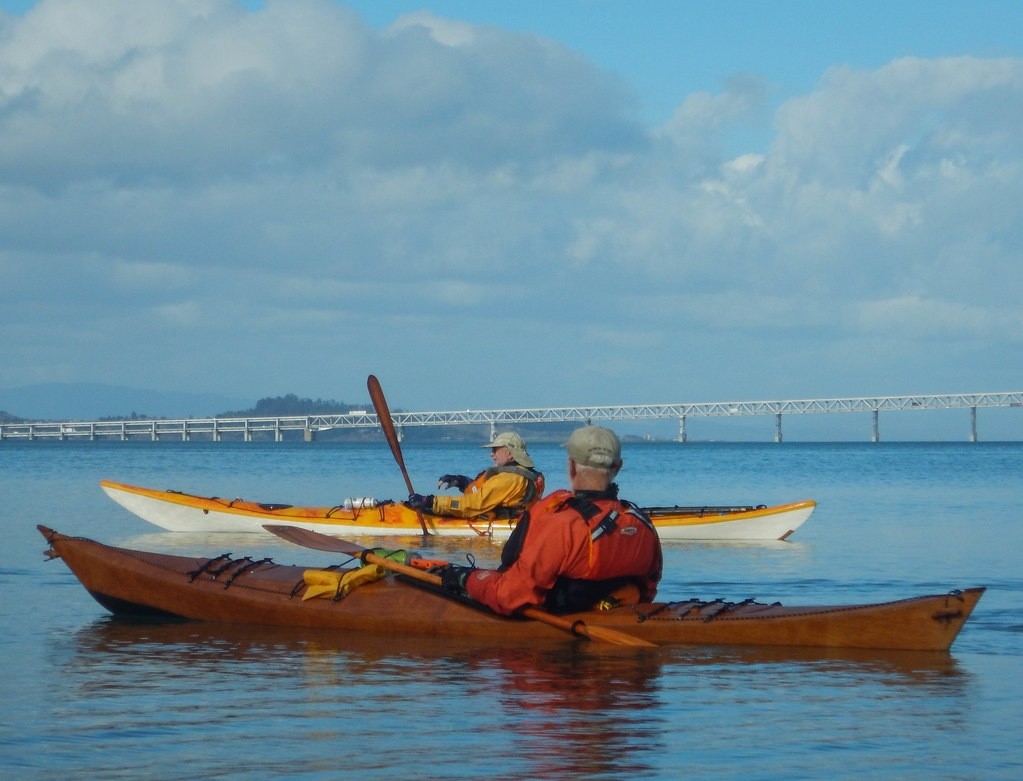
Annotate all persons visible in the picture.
[409,432,534,519]
[420,425,663,617]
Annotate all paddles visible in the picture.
[365,372,436,547]
[259,520,657,650]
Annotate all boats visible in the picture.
[99,480,817,540]
[37,524,987,652]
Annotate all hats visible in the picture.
[481,431,534,468]
[559,425,621,469]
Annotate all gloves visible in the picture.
[408,494,435,508]
[437,474,469,490]
[434,553,479,597]
